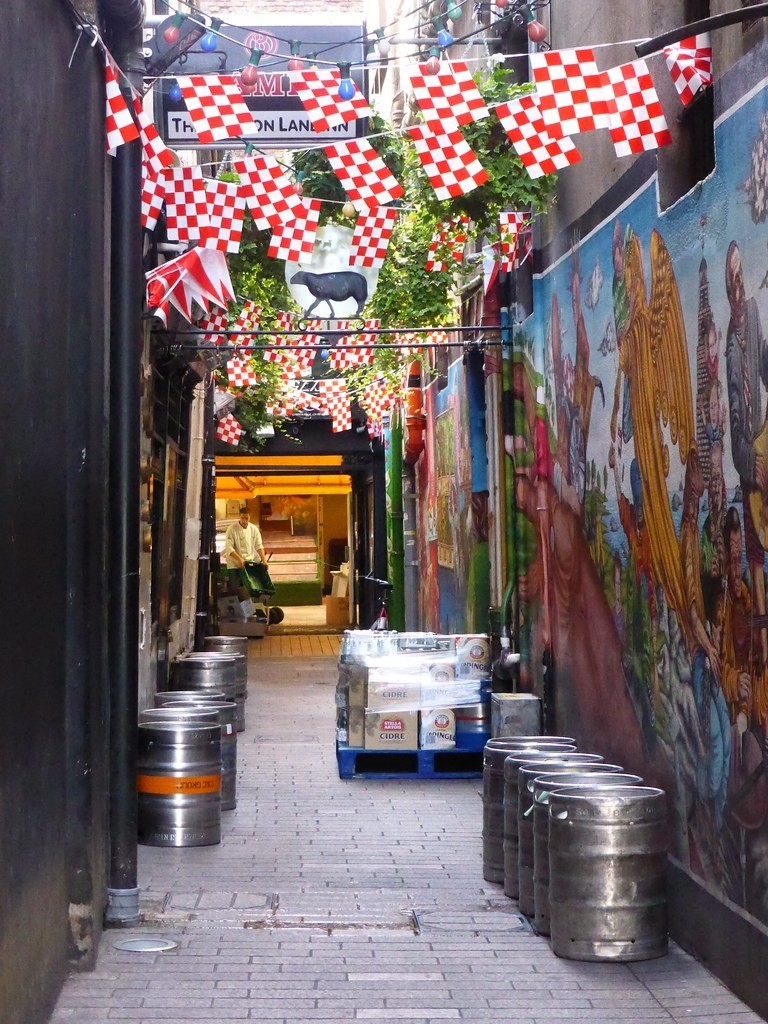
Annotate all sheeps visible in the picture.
[290,271,369,318]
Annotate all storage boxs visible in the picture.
[338,635,491,751]
[218,559,277,637]
[326,596,349,627]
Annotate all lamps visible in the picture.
[213,389,237,421]
[201,341,231,372]
[169,319,203,350]
[142,13,206,85]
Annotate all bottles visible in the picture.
[334,627,445,744]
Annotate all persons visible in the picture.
[225,507,269,566]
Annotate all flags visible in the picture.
[141,165,449,445]
[662,31,714,106]
[597,59,672,158]
[408,116,490,201]
[198,178,244,254]
[131,84,176,179]
[405,61,490,137]
[286,68,376,134]
[175,75,259,144]
[163,166,203,240]
[324,137,405,212]
[105,49,141,157]
[482,212,533,297]
[494,93,582,179]
[425,214,469,272]
[530,49,612,139]
[234,155,307,231]
[349,206,397,269]
[267,196,321,264]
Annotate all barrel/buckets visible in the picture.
[481,736,668,964]
[136,636,249,848]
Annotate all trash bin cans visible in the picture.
[490,693,541,739]
